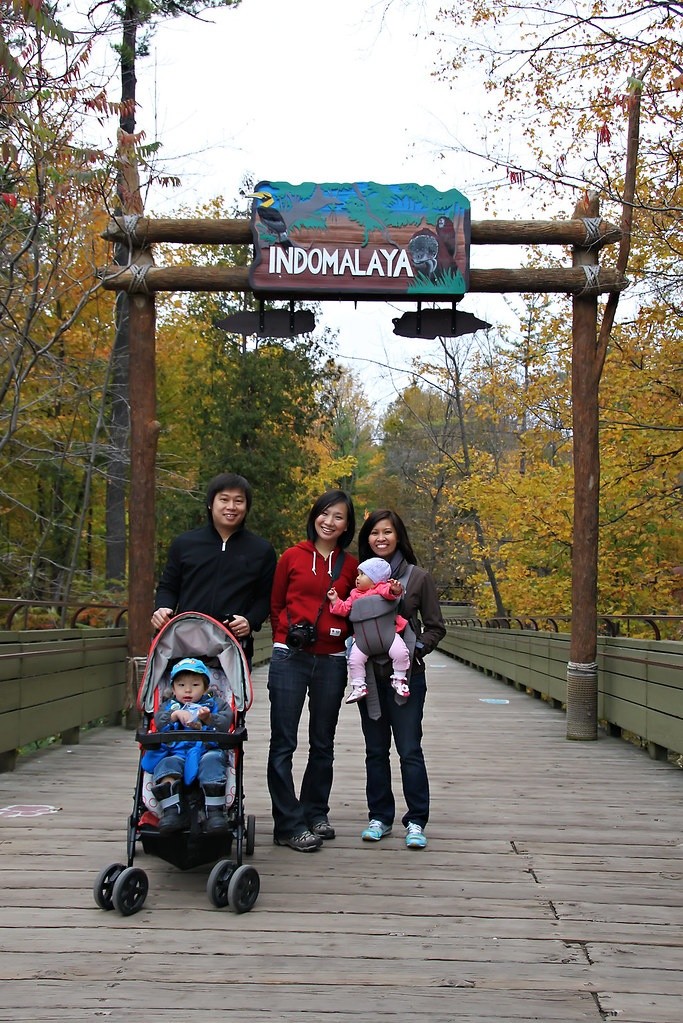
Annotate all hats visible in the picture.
[357,557,391,585]
[169,657,211,686]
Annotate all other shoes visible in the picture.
[389,674,410,696]
[345,682,369,704]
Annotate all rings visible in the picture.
[237,630,240,634]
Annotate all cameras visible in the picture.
[286,623,316,651]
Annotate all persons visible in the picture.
[153,658,234,833]
[134,472,278,840]
[267,489,360,853]
[356,510,446,848]
[327,556,412,705]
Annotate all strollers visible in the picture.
[93,607,260,917]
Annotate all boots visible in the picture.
[150,778,192,834]
[203,779,228,833]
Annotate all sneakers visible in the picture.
[405,821,428,848]
[310,821,337,840]
[362,818,393,841]
[274,827,323,853]
